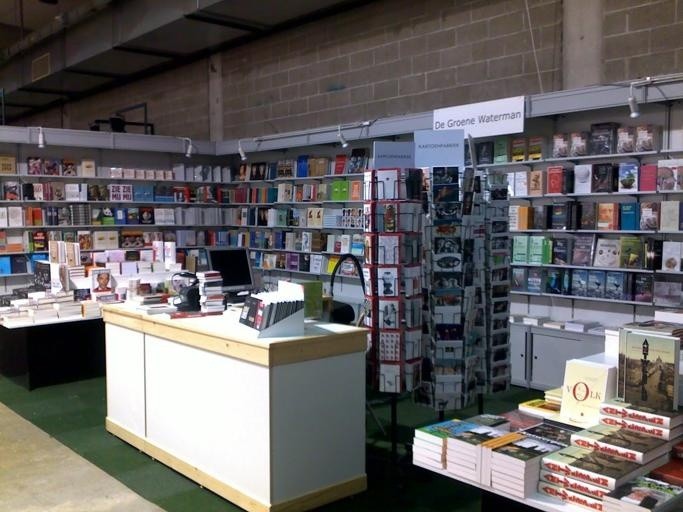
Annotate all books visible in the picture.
[1,147,374,331]
[376,122,683,511]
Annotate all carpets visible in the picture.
[0,374,546,512]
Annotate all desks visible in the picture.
[99,295,369,512]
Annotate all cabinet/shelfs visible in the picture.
[509,327,532,389]
[229,144,683,313]
[359,165,427,464]
[529,333,605,391]
[333,295,366,326]
[0,171,228,280]
[413,164,489,426]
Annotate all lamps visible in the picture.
[336,119,377,148]
[178,136,193,158]
[36,125,47,149]
[238,139,249,161]
[626,76,655,119]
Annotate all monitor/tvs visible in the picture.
[205,245,256,303]
